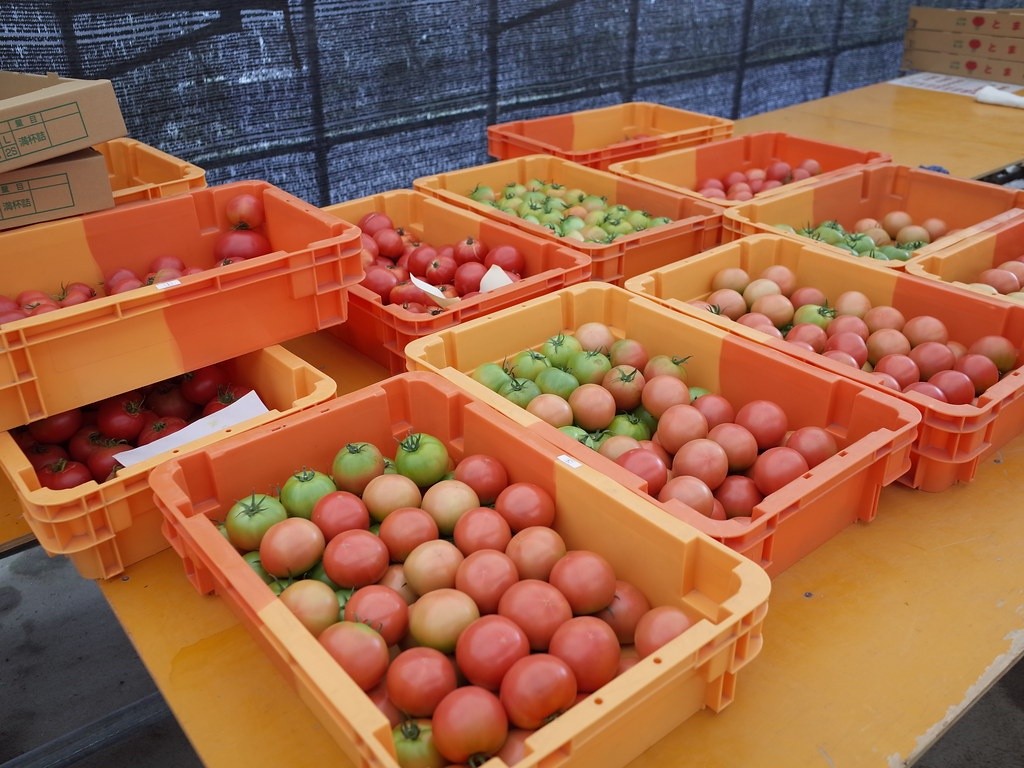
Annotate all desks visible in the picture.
[101,79,1023,768]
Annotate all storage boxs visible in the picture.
[489,102,737,174]
[412,155,728,287]
[148,372,774,768]
[615,162,1024,490]
[901,7,1024,84]
[314,192,595,377]
[610,130,891,214]
[406,278,921,582]
[0,69,365,577]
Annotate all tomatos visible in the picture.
[693,158,823,201]
[352,212,526,313]
[686,265,1017,408]
[470,321,838,521]
[0,193,271,326]
[7,363,257,489]
[772,209,1024,300]
[215,429,694,768]
[467,178,678,248]
